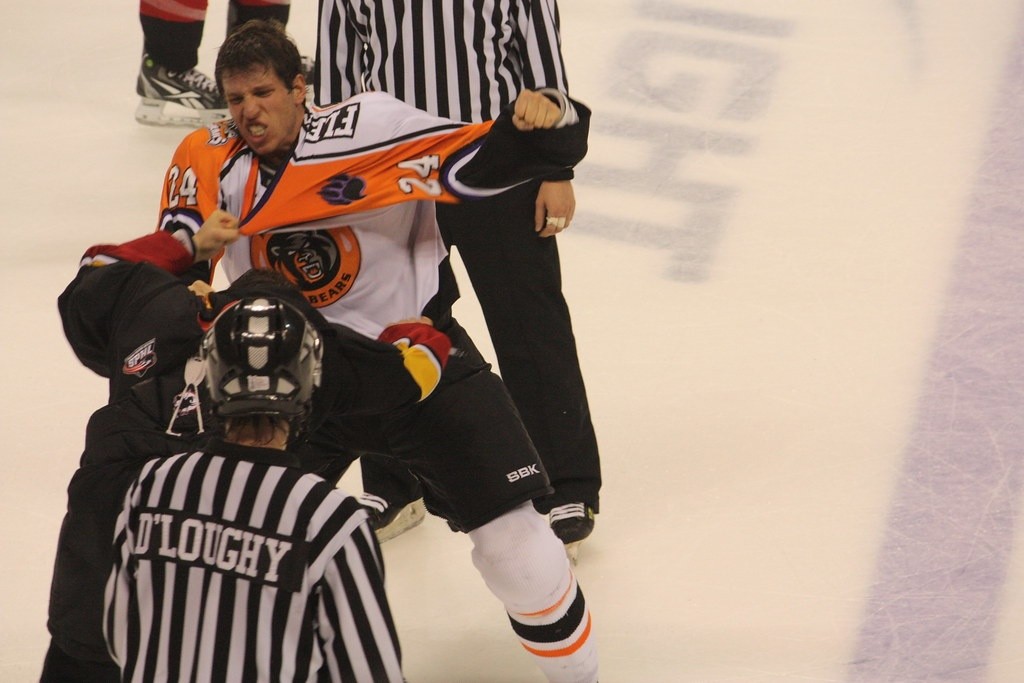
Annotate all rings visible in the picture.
[558,217,566,227]
[546,217,558,225]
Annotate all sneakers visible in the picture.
[301,53,317,97]
[548,501,597,565]
[374,488,429,544]
[135,54,233,131]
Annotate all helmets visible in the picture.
[206,266,334,417]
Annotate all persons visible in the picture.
[157,18,602,683]
[134,0,316,128]
[312,0,602,566]
[102,293,407,683]
[40,208,453,683]
